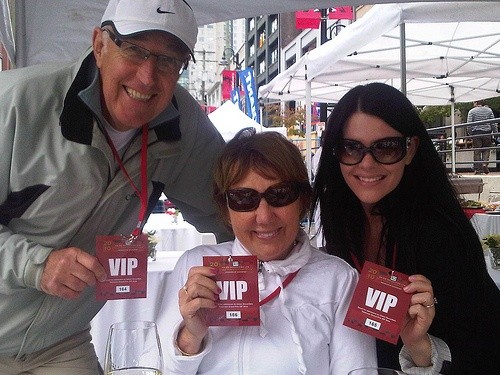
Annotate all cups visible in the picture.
[488,191,500,210]
[348,367,407,375]
[105,321,163,375]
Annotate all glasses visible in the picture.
[335,136,412,165]
[102,28,191,75]
[225,180,301,212]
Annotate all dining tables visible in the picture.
[462,202,500,256]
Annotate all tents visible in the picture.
[257,0,500,188]
[208,100,288,143]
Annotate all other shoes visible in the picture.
[483,165,489,174]
[475,170,482,175]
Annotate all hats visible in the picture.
[100,0,199,63]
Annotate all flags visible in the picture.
[236,67,260,124]
[230,87,242,111]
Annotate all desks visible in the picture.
[140,213,216,251]
[87,250,180,371]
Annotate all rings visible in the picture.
[183,286,188,294]
[426,297,438,307]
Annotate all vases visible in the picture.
[488,249,500,270]
[170,215,178,224]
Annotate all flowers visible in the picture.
[164,200,178,215]
[480,233,500,248]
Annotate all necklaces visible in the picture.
[294,240,297,247]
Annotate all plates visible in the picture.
[483,208,500,213]
[460,202,486,208]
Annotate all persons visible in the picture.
[308,83,500,375]
[139,127,377,375]
[466,100,498,175]
[0,0,235,375]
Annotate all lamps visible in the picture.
[219,47,242,69]
